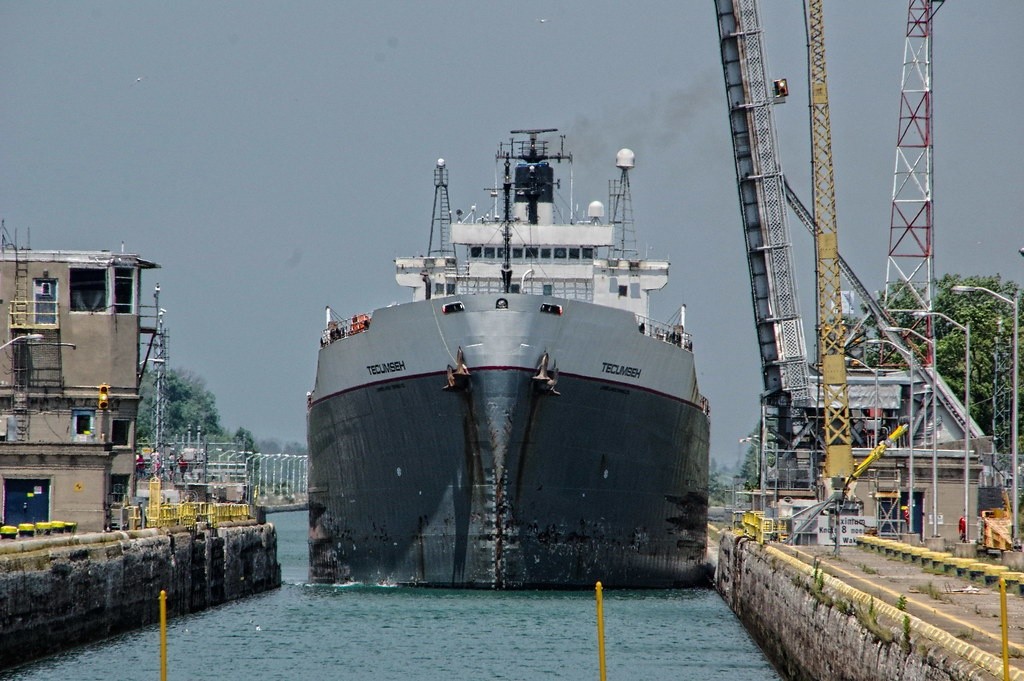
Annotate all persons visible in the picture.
[136,455,146,479]
[150,448,159,477]
[168,452,175,474]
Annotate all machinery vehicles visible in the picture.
[770,0,945,555]
[731,423,909,545]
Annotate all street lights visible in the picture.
[844,312,978,558]
[950,285,1024,575]
[218,449,308,499]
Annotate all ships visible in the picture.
[306,128,712,588]
